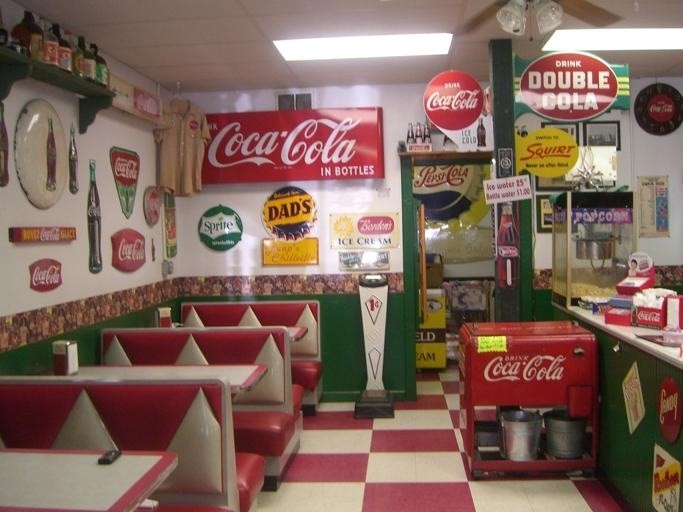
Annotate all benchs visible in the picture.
[96,328,291,492]
[0,378,241,512]
[291,383,304,449]
[178,303,323,415]
[234,448,265,512]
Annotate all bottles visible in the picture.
[44,116,57,191]
[163,191,178,259]
[0,102,10,188]
[85,157,102,274]
[476,116,486,147]
[423,121,431,144]
[493,203,521,292]
[414,121,423,144]
[66,124,79,196]
[9,8,109,86]
[406,121,415,145]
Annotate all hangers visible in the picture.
[158,77,192,115]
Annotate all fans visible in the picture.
[454,0,622,38]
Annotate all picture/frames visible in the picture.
[531,120,621,233]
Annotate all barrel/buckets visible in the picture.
[12,11,109,86]
[496,411,544,463]
[543,410,588,459]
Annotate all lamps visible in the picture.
[494,0,564,35]
